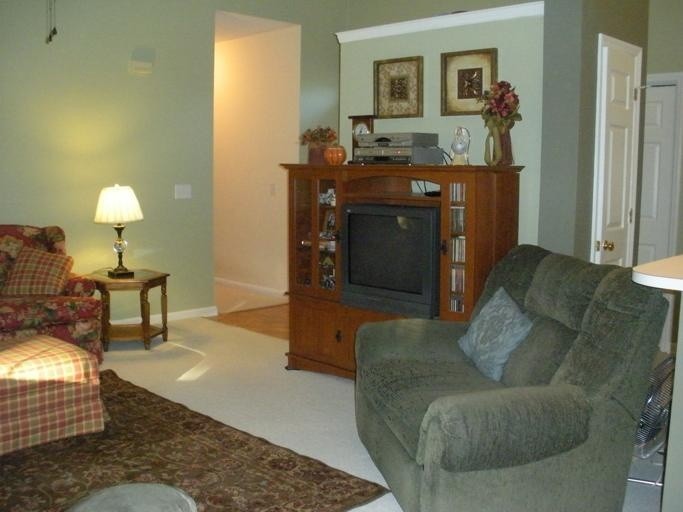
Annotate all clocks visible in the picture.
[347,115,376,161]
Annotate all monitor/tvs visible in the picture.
[340,203,439,320]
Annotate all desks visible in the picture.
[630,253,683,512]
[84,266,170,348]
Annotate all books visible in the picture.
[448,183,465,313]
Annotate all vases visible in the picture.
[482,124,511,167]
[308,145,327,165]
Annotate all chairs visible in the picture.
[0,222,108,368]
[352,241,667,511]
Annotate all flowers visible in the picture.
[483,84,522,124]
[299,127,336,149]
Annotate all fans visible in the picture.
[625,353,675,487]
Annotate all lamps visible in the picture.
[92,183,142,278]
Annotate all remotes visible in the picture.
[424,191,440,197]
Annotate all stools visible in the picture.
[0,330,107,460]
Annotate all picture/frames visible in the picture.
[371,57,423,119]
[439,49,497,115]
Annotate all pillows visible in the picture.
[3,246,72,295]
[457,285,531,381]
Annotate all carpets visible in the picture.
[0,370,392,510]
[201,303,289,340]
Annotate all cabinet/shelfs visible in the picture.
[283,292,405,381]
[279,161,522,324]
